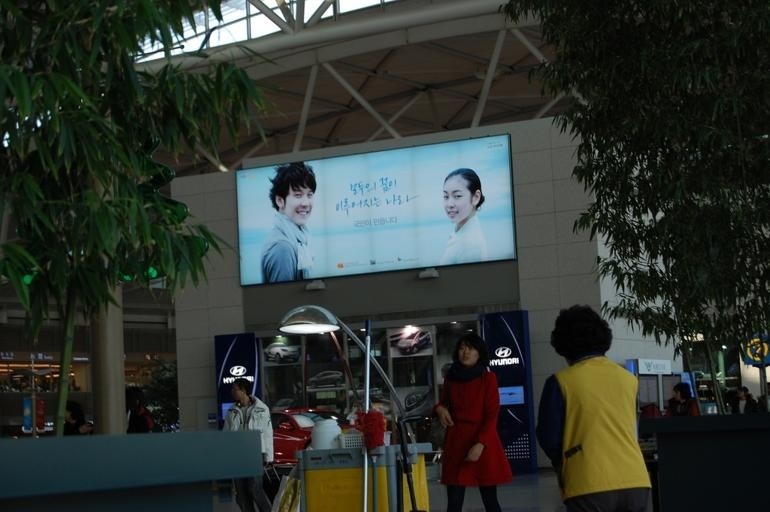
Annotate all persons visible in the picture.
[221,378,277,511]
[435,167,485,263]
[428,331,514,510]
[732,386,757,414]
[533,303,653,510]
[62,399,90,435]
[258,162,317,283]
[666,382,699,416]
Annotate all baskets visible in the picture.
[339,431,392,447]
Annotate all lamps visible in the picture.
[274,303,417,442]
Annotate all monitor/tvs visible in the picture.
[636,373,660,412]
[661,373,681,412]
[498,385,525,405]
[222,402,235,420]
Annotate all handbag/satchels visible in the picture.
[431,416,448,450]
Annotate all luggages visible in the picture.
[262,462,289,504]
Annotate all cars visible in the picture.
[307,370,344,388]
[263,340,300,365]
[269,405,366,464]
[397,329,431,355]
